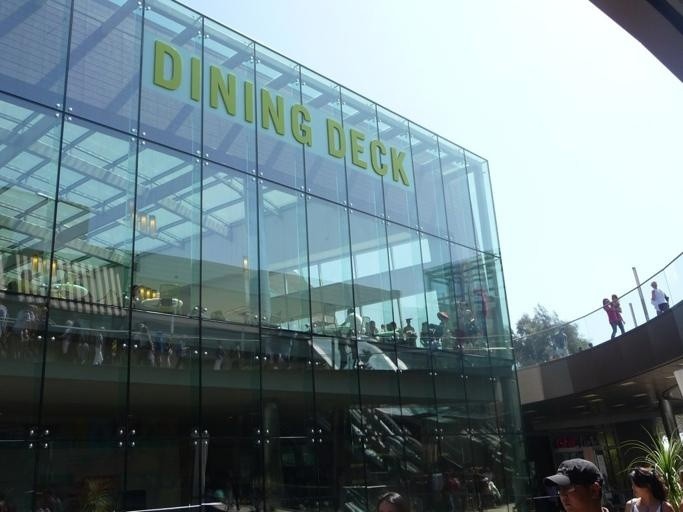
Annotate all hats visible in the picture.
[541,457,602,488]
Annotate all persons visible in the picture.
[377,492,410,512]
[650,280,669,316]
[543,458,609,512]
[611,295,626,324]
[623,466,683,512]
[481,477,501,506]
[342,308,365,334]
[447,471,463,512]
[603,298,625,339]
